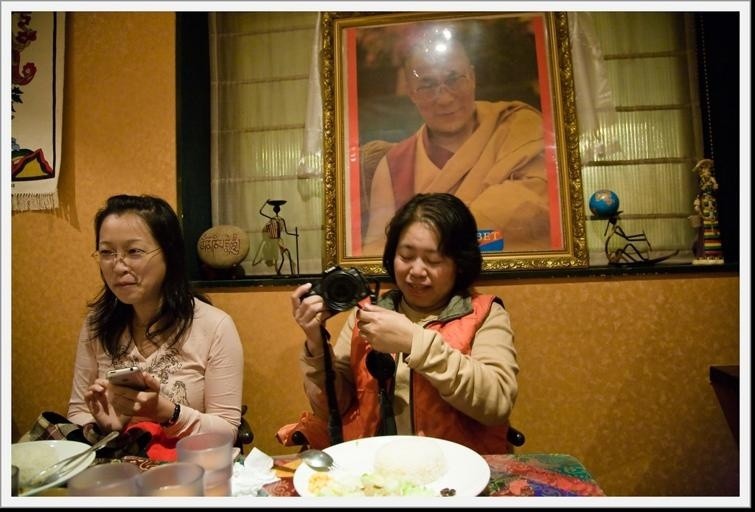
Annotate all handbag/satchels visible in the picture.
[17,411,151,463]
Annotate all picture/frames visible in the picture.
[322,14,591,277]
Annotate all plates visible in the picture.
[293,435,492,498]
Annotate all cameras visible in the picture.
[302,262,379,313]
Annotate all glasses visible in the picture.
[90,247,161,266]
[414,69,473,103]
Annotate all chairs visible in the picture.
[290,427,531,462]
[233,405,254,454]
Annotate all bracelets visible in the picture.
[161,405,180,428]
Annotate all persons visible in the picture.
[68,195,244,450]
[364,28,550,254]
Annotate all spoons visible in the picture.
[270,450,333,468]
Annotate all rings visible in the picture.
[315,315,321,321]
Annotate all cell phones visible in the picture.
[107,367,147,392]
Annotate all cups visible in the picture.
[10,431,233,497]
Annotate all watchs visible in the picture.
[290,192,520,457]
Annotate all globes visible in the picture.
[589,190,651,264]
